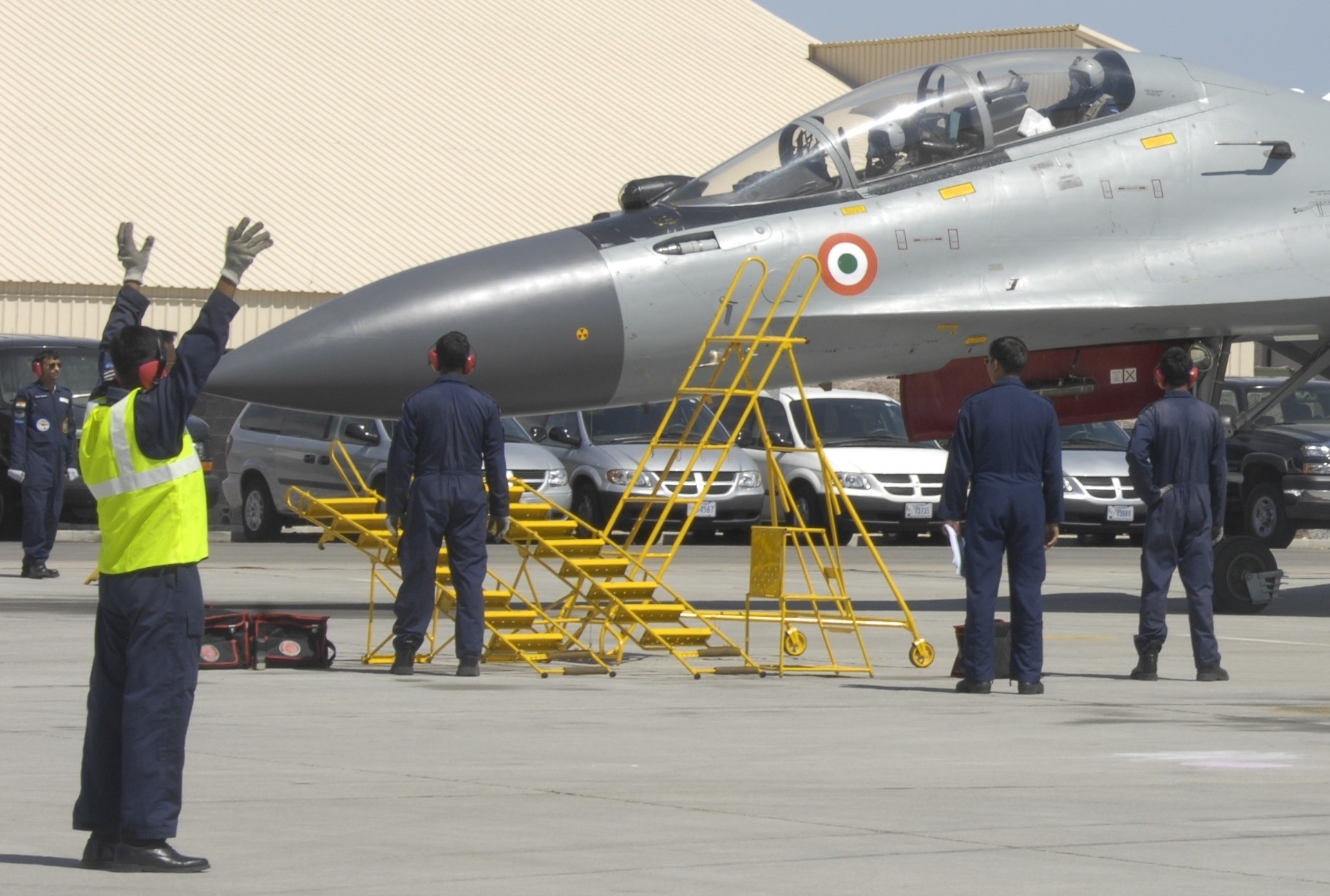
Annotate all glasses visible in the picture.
[43,362,62,369]
[984,358,997,364]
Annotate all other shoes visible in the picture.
[390,656,414,674]
[457,659,481,676]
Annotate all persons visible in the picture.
[72,216,278,873]
[1054,56,1120,129]
[7,350,79,577]
[383,331,511,676]
[1125,345,1230,682]
[857,404,886,439]
[864,121,916,181]
[939,336,1065,695]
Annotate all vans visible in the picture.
[220,400,574,545]
[1041,420,1150,546]
[512,396,765,544]
[694,387,972,546]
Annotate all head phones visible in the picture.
[1153,365,1200,389]
[428,350,476,376]
[32,358,44,377]
[136,328,169,391]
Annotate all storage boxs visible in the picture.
[954,619,1013,679]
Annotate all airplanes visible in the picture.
[200,50,1330,420]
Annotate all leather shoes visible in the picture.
[80,834,113,867]
[47,569,60,577]
[112,842,210,872]
[956,679,992,695]
[1197,662,1229,681]
[1018,681,1045,694]
[21,564,49,577]
[1130,653,1158,682]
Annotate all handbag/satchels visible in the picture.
[952,618,1012,679]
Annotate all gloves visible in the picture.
[488,515,511,541]
[219,217,274,285]
[64,467,79,482]
[117,222,155,285]
[384,513,404,538]
[7,469,26,484]
[1211,526,1223,545]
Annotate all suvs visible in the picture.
[0,332,221,526]
[1215,375,1330,549]
